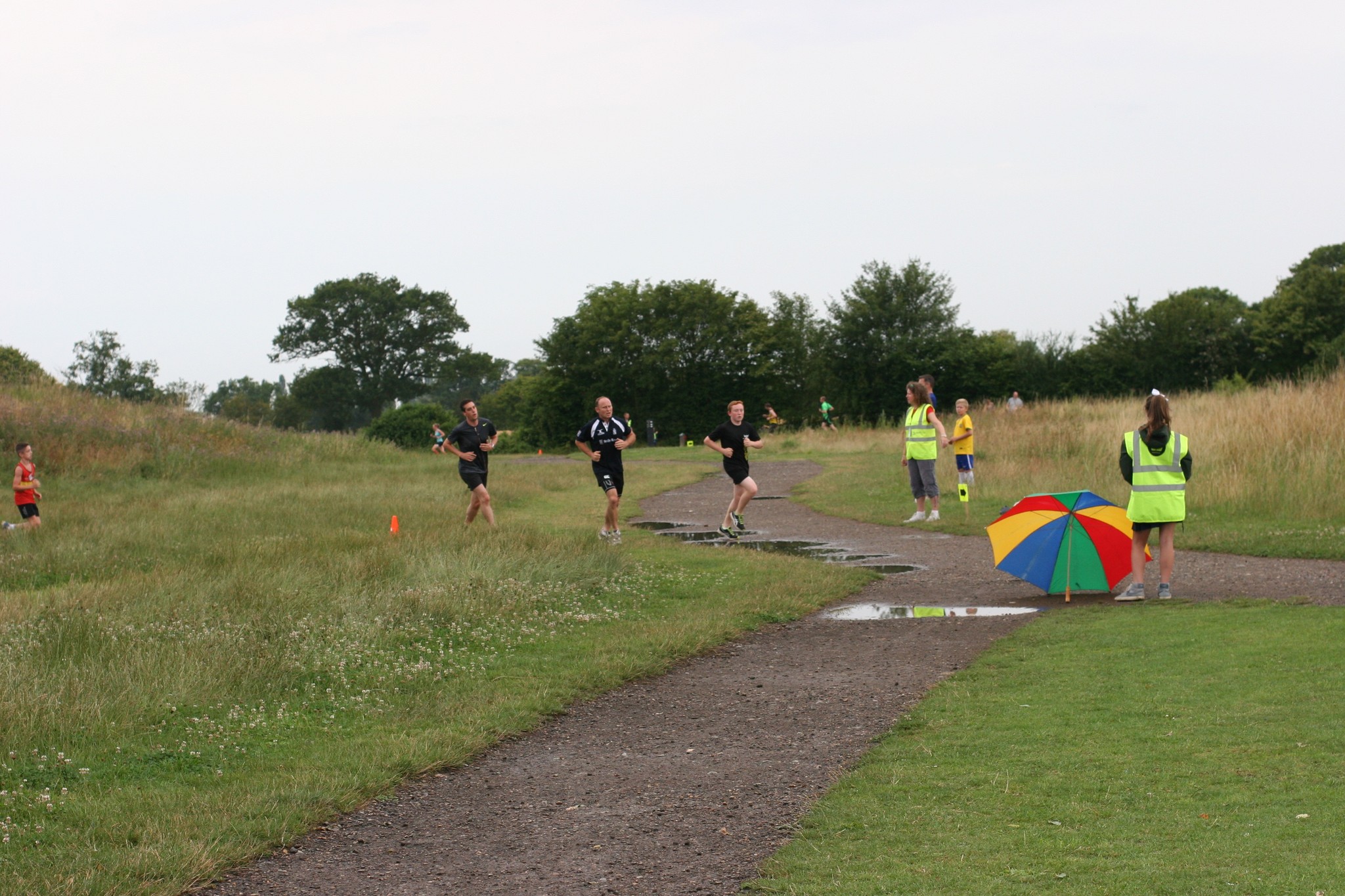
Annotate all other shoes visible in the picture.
[2,521,10,530]
[613,530,622,545]
[597,529,611,541]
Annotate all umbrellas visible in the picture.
[980,488,1154,604]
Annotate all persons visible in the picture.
[623,412,633,430]
[1111,388,1195,602]
[653,430,659,446]
[917,375,937,412]
[701,399,765,539]
[441,399,500,529]
[897,381,950,525]
[816,396,840,433]
[1006,392,1024,413]
[947,398,976,486]
[573,395,639,545]
[761,403,779,437]
[430,422,445,455]
[983,397,996,414]
[2,442,43,530]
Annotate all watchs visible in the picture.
[940,434,948,438]
[491,442,496,450]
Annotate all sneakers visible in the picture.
[903,513,926,523]
[717,524,740,540]
[1114,583,1145,600]
[926,513,940,521]
[730,511,746,531]
[1158,583,1171,599]
